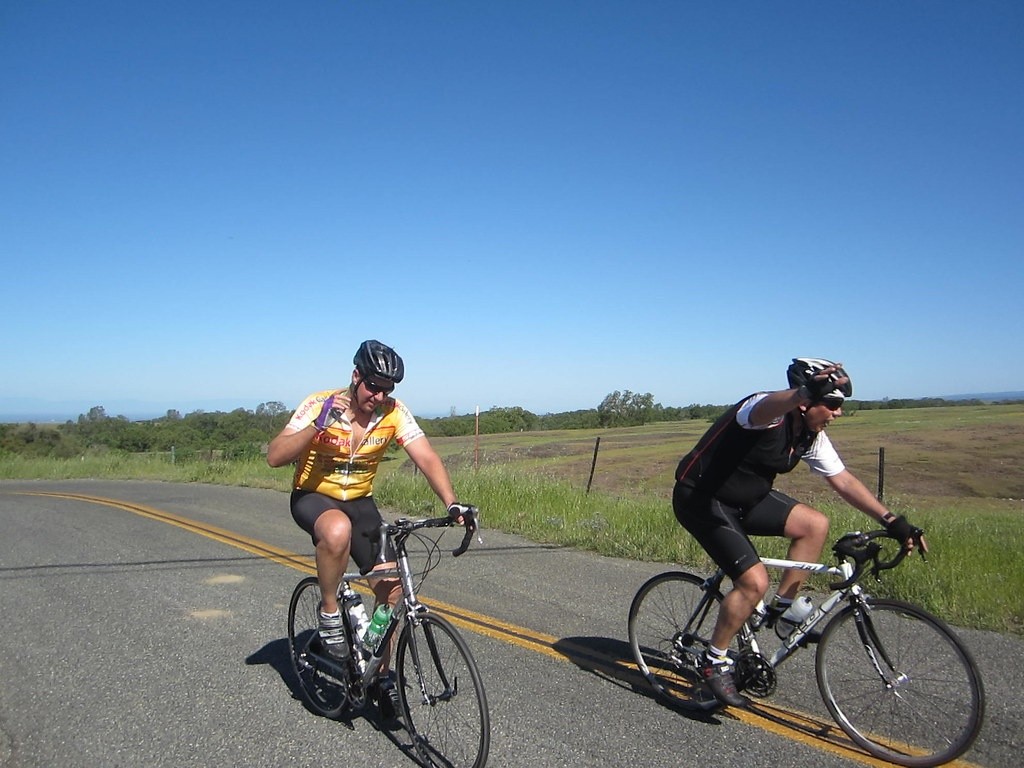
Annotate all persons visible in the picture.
[268,339,479,715]
[671,357,927,707]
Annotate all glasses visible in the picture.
[820,398,843,411]
[356,366,394,395]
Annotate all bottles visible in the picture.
[344,589,370,644]
[750,599,767,627]
[773,595,813,640]
[363,603,392,646]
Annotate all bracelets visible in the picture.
[881,512,895,526]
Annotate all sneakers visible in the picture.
[701,650,747,707]
[369,675,404,717]
[316,600,349,661]
[765,597,820,643]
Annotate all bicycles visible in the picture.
[626,524,985,768]
[286,505,492,768]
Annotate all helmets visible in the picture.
[353,340,404,383]
[787,357,852,401]
